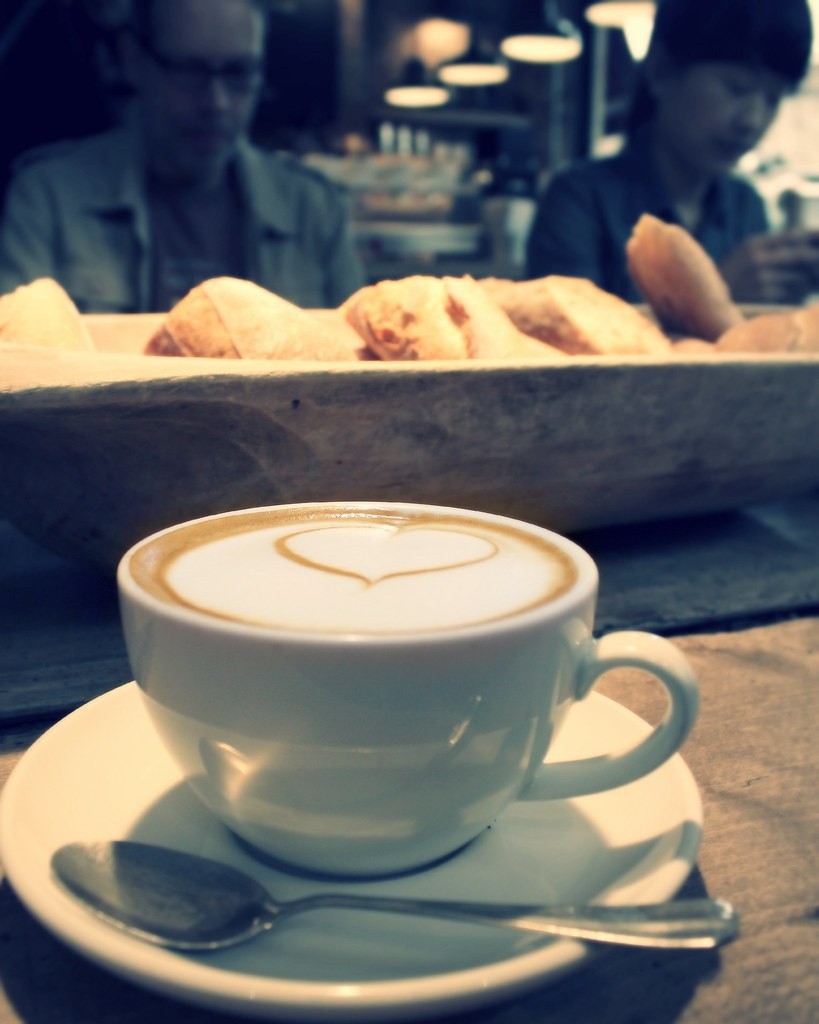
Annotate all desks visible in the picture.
[1,493,819,1024]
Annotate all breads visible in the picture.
[0,211,819,364]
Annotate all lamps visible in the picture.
[582,0,660,28]
[384,57,450,108]
[499,1,582,64]
[436,34,509,86]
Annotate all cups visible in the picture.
[116,501,700,881]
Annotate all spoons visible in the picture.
[51,841,736,949]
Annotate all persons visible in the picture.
[526,0,818,306]
[1,0,363,309]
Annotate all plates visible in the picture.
[0,679,704,1024]
